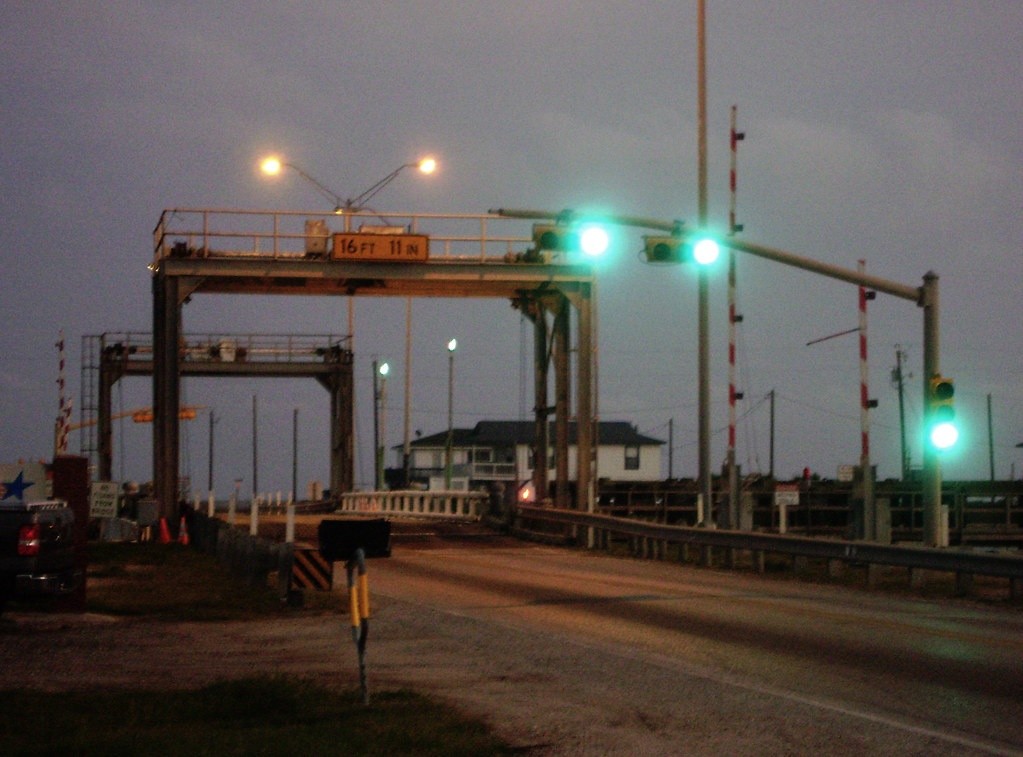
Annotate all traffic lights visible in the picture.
[641,233,719,268]
[533,225,605,253]
[928,374,956,448]
[132,414,154,422]
[179,411,196,420]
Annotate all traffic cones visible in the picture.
[159,517,174,545]
[178,517,190,546]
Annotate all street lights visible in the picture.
[379,363,390,490]
[445,338,458,489]
[258,155,439,488]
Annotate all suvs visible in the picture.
[0,500,89,609]
